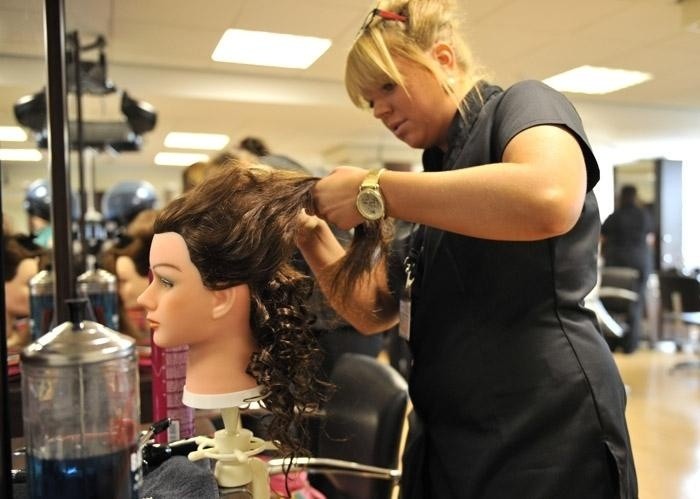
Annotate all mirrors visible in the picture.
[2,2,76,498]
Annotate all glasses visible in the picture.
[353,8,408,36]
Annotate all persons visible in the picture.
[2,239,40,339]
[600,180,656,353]
[299,0,638,498]
[115,238,150,311]
[134,158,388,413]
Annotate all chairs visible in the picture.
[266,353,410,499]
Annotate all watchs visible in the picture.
[355,166,387,221]
